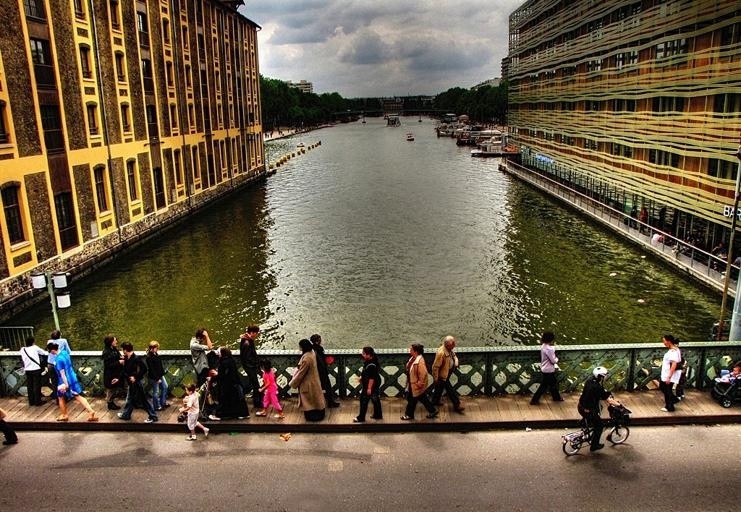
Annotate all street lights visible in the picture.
[30,266,72,335]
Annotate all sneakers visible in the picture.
[432,401,444,407]
[453,404,466,412]
[399,415,415,420]
[677,394,685,400]
[203,427,210,438]
[53,415,69,422]
[186,434,197,441]
[4,435,19,443]
[87,411,97,421]
[208,402,286,423]
[589,443,606,452]
[352,417,365,422]
[327,402,341,408]
[660,406,675,412]
[105,397,171,424]
[426,411,440,419]
[370,414,383,420]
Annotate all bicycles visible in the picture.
[562,401,630,457]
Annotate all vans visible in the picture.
[445,114,457,121]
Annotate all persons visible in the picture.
[629,206,741,284]
[400,343,439,420]
[352,347,383,421]
[101,335,172,423]
[429,335,465,412]
[578,367,620,453]
[0,408,18,444]
[529,331,564,405]
[178,325,341,440]
[21,331,99,421]
[658,334,741,412]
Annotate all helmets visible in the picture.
[591,366,608,379]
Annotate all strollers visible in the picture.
[711,370,741,408]
[177,375,215,421]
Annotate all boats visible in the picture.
[406,133,416,141]
[385,116,402,127]
[384,115,390,120]
[298,142,305,147]
[435,122,522,157]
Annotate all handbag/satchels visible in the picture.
[668,361,684,371]
